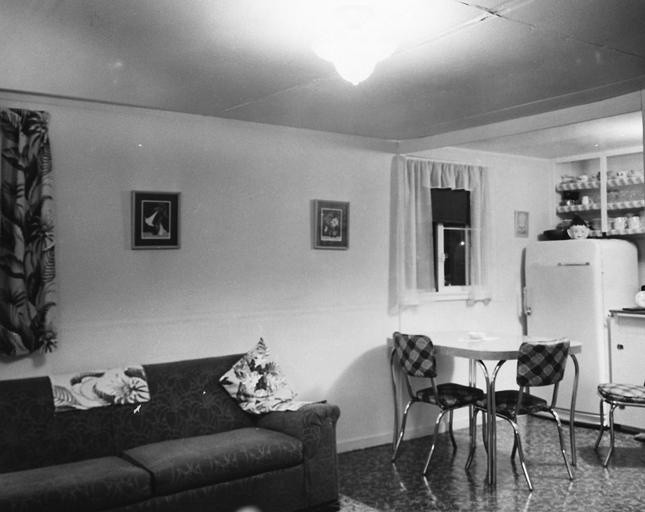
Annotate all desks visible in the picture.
[392,336,582,484]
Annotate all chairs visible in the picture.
[391,332,487,476]
[465,339,574,491]
[594,383,645,468]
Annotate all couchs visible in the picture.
[0,354,341,511]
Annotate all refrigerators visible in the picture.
[522,238,641,429]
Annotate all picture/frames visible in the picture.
[131,190,180,250]
[315,200,349,250]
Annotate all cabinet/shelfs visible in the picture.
[553,145,644,236]
